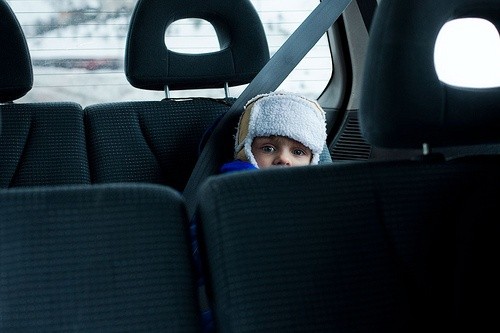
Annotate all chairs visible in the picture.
[0,0,91,190]
[194,0,500,333]
[84,0,271,220]
[0,182,201,333]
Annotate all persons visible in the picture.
[234,92,327,170]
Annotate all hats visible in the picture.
[233,92,327,169]
